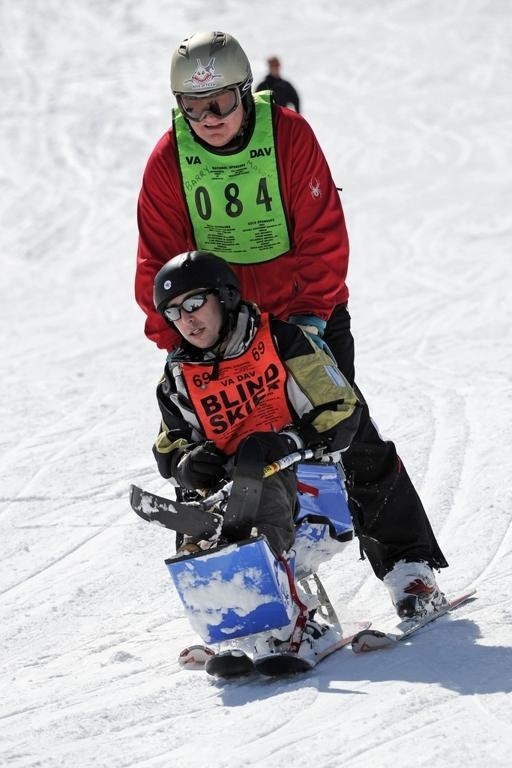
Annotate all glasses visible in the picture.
[174,80,253,122]
[162,288,214,321]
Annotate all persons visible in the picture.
[255,55,300,113]
[150,249,365,677]
[133,29,450,663]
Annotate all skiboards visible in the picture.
[179,642,219,670]
[352,588,475,654]
[178,588,478,676]
[206,616,372,675]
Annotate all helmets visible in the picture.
[170,30,251,93]
[152,249,240,312]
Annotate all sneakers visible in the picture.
[383,561,444,615]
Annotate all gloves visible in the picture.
[233,431,298,466]
[170,438,229,489]
